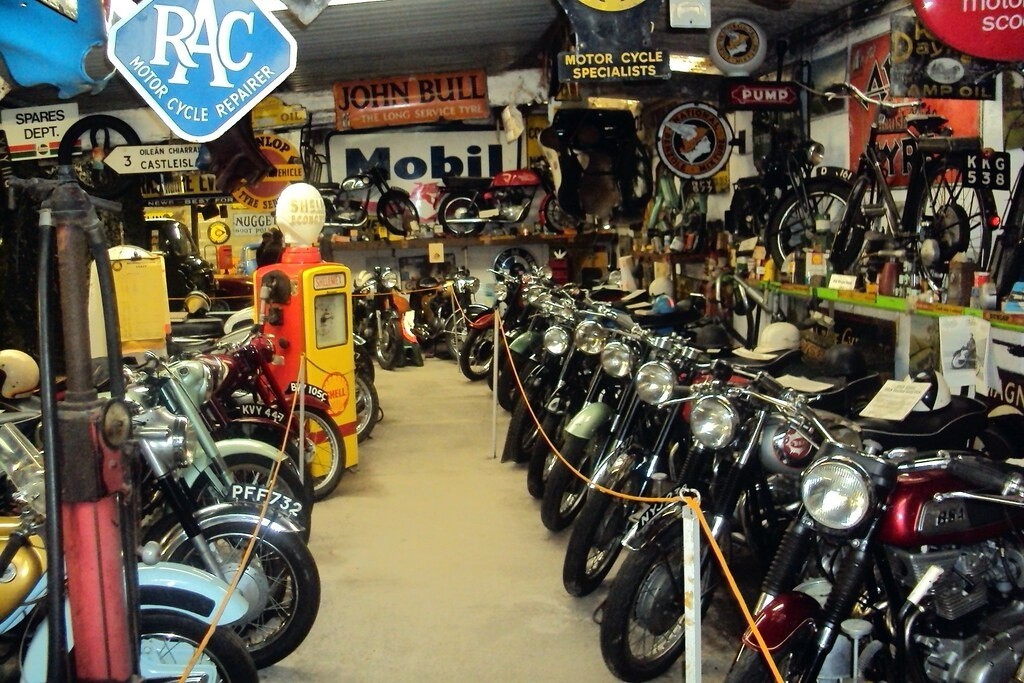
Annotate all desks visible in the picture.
[168,274,254,300]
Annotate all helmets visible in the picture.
[0,348,41,403]
[905,369,951,412]
[754,321,801,354]
[821,343,866,377]
[690,326,729,350]
[649,277,674,298]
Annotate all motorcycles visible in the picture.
[725,105,866,269]
[433,154,585,237]
[0,269,1024,683]
[313,161,422,240]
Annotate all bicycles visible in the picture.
[827,81,1000,298]
